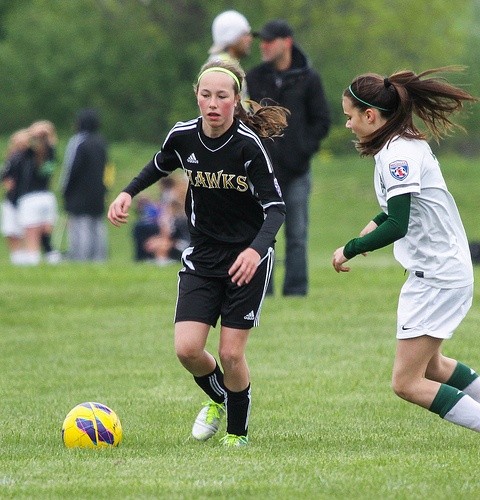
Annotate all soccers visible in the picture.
[61,400,122,448]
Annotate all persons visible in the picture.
[0,120,58,265]
[208,11,253,113]
[58,111,109,261]
[132,176,190,262]
[106,59,292,446]
[331,65,480,432]
[250,20,332,296]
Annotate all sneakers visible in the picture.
[218,432,249,448]
[189,399,225,441]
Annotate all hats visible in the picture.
[206,10,251,54]
[253,20,292,39]
[76,109,98,130]
